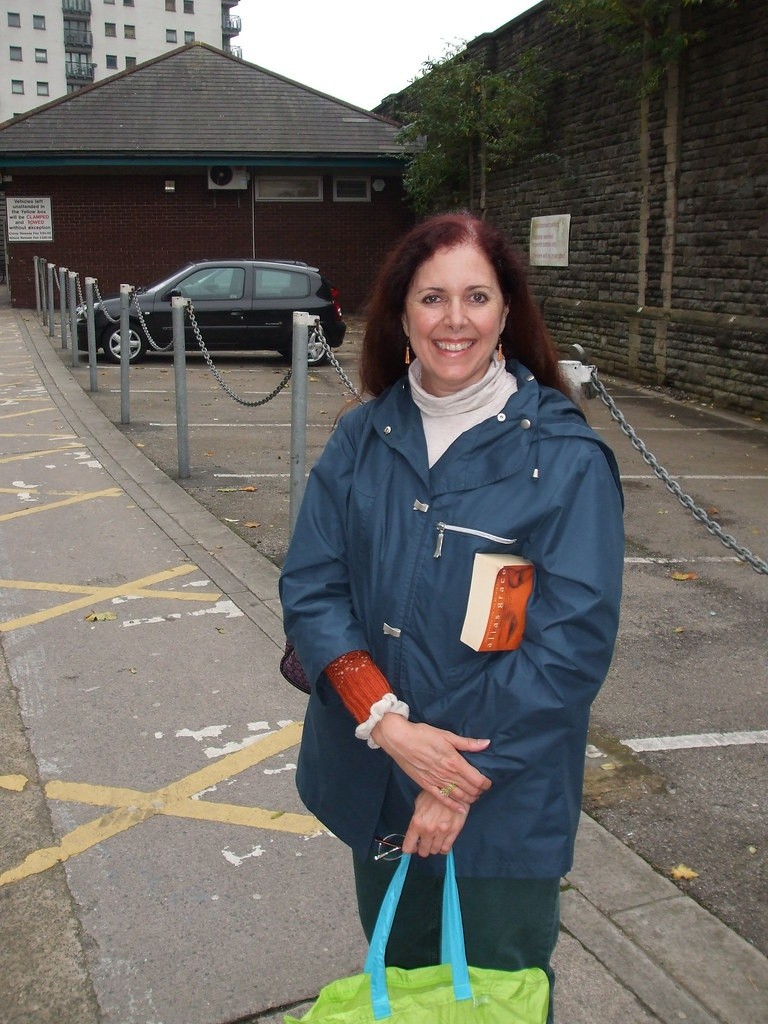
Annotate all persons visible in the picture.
[279,210,628,1023]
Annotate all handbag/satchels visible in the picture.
[282,844,552,1024]
[280,635,311,694]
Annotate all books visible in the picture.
[460,549,536,651]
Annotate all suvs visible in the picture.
[68,260,346,367]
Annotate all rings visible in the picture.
[439,783,457,797]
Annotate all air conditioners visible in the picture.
[208,164,251,189]
